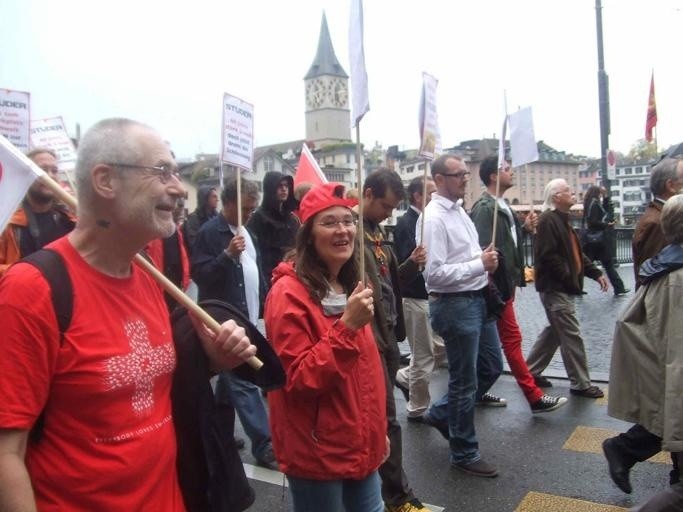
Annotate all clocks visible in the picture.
[306,79,327,109]
[327,78,349,108]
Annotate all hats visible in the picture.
[298,183,359,223]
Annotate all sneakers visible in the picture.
[532,394,568,413]
[385,497,431,512]
[396,380,449,441]
[532,377,552,387]
[234,436,279,469]
[615,289,630,295]
[451,459,499,476]
[474,392,507,407]
[570,386,604,398]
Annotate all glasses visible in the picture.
[314,218,358,226]
[440,172,470,178]
[104,162,181,184]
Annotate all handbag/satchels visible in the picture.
[581,230,607,258]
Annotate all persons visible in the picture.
[1,116,683,512]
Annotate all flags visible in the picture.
[644,73,658,138]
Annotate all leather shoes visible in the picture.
[603,439,631,494]
